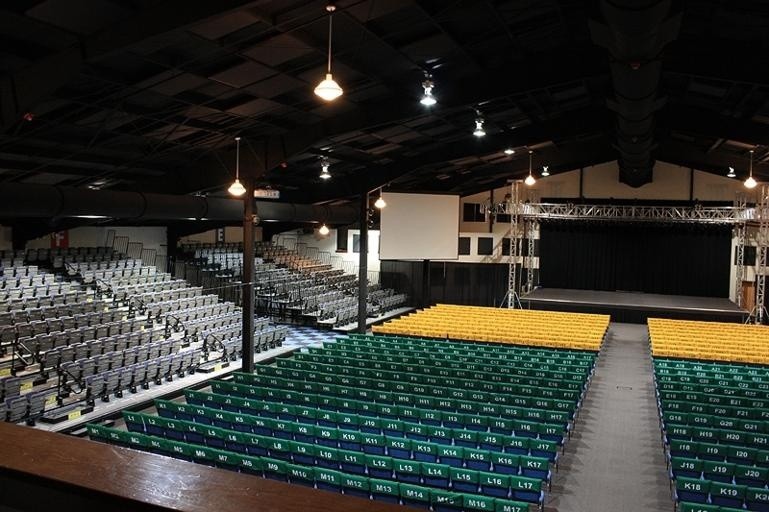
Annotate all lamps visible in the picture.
[227,139,247,198]
[313,7,343,102]
[319,71,550,235]
[744,151,758,189]
[727,163,737,177]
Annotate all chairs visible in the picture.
[0,241,611,512]
[647,317,769,512]
